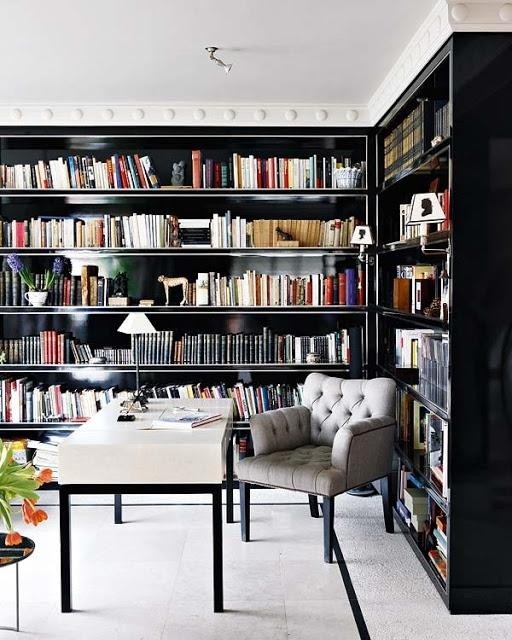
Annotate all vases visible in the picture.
[23,292,48,307]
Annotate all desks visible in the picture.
[55,395,235,615]
[0,531,35,632]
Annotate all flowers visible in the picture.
[8,252,63,292]
[0,439,53,547]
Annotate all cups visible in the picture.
[23,291,49,306]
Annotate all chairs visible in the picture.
[237,371,399,565]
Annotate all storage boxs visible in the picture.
[403,488,428,533]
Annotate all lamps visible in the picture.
[349,224,375,266]
[205,45,232,74]
[404,190,451,259]
[117,310,157,404]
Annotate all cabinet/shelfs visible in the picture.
[373,32,510,615]
[1,126,376,492]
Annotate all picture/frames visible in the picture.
[406,399,431,453]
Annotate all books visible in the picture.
[0,327,363,365]
[0,265,128,306]
[209,212,362,247]
[0,150,349,189]
[239,437,253,455]
[381,94,449,588]
[0,213,172,248]
[1,377,304,423]
[151,406,223,429]
[33,432,70,478]
[185,265,363,305]
